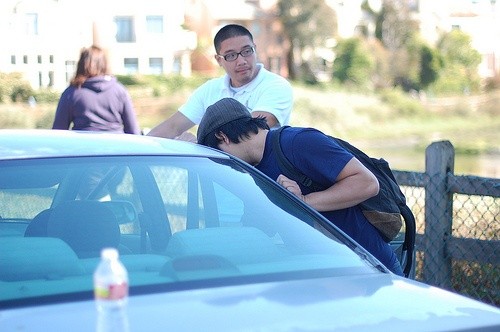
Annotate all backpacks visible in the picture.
[273,125,407,242]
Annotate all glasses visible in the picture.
[218,44,256,61]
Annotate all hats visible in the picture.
[196,97,252,144]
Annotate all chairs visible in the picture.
[1,199,292,280]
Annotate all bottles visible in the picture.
[93,248,128,332]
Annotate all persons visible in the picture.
[50,44,140,203]
[196,98,406,281]
[146,25,293,144]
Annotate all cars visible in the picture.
[1,129,500,332]
